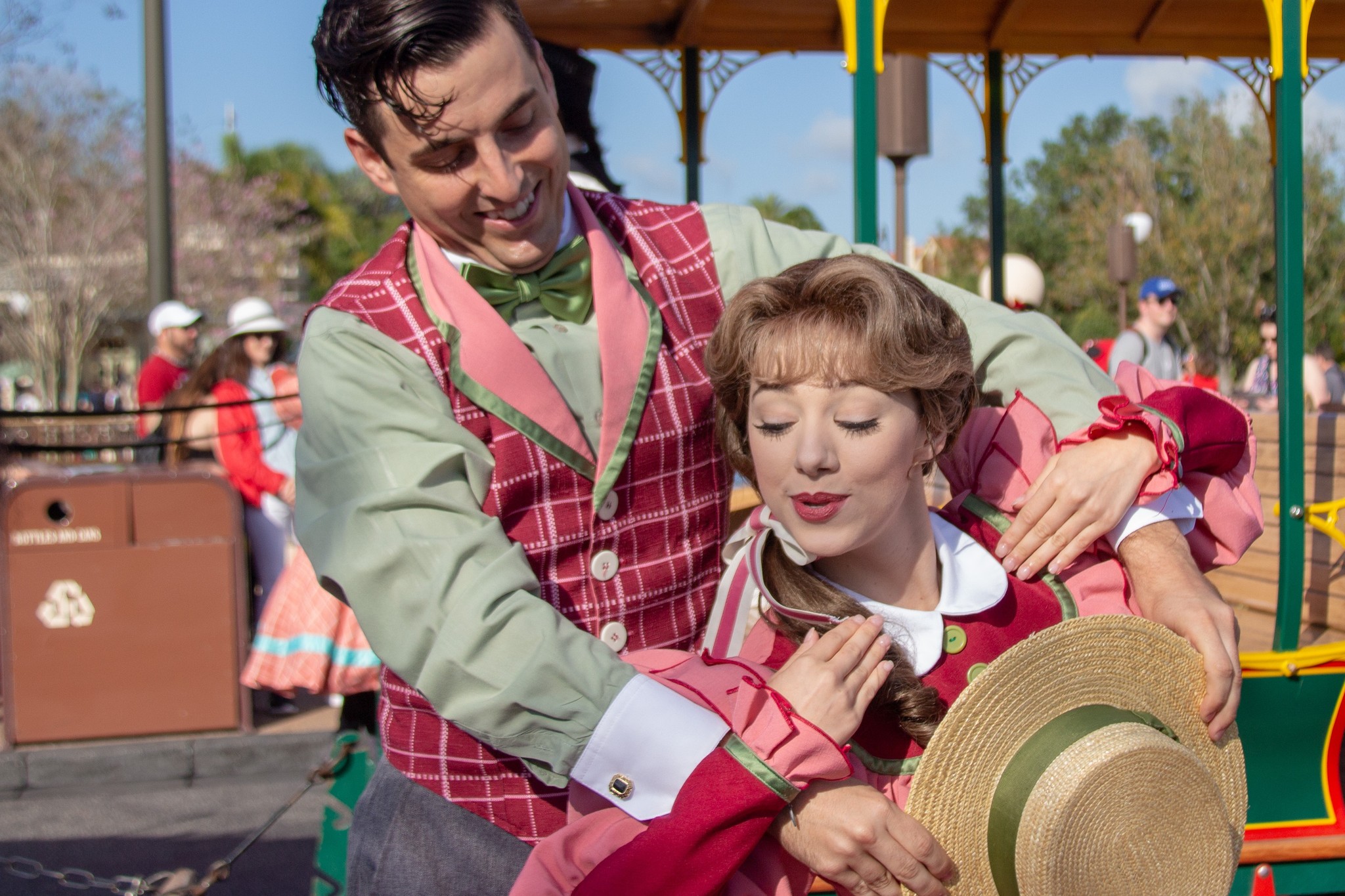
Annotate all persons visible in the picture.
[1181,340,1218,391]
[1108,277,1185,381]
[293,0,1240,896]
[1244,316,1331,407]
[243,370,380,734]
[507,253,1264,896]
[166,346,228,468]
[1313,346,1345,405]
[215,298,300,715]
[138,302,206,462]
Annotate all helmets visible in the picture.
[977,255,1046,310]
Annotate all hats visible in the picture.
[901,616,1248,896]
[148,300,202,337]
[1140,277,1186,304]
[225,298,289,337]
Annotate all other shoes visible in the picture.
[267,691,298,714]
[321,692,344,708]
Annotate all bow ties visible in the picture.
[460,235,592,325]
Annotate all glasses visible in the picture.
[1261,337,1279,345]
[1157,297,1178,305]
